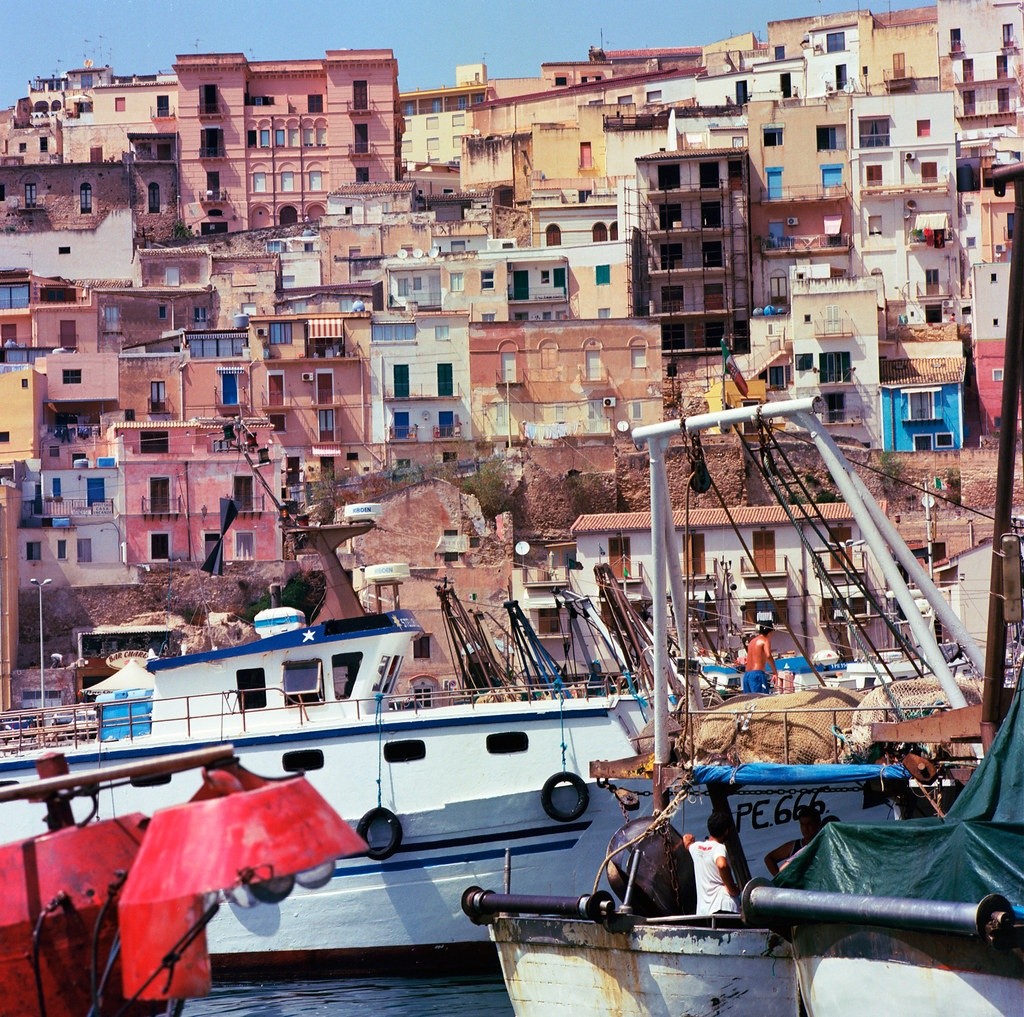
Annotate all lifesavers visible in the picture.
[541,771,590,822]
[354,807,405,863]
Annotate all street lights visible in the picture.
[29,579,53,709]
[825,540,866,645]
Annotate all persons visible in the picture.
[743,620,779,695]
[682,812,743,930]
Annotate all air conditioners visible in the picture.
[302,372,315,383]
[255,328,268,337]
[787,217,799,226]
[603,397,617,408]
[942,299,954,309]
[995,244,1007,254]
[507,263,514,273]
[905,152,915,161]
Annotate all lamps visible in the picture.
[280,504,291,523]
[222,423,271,463]
[201,505,208,524]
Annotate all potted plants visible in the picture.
[910,228,921,238]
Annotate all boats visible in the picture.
[1,396,1024,1017]
